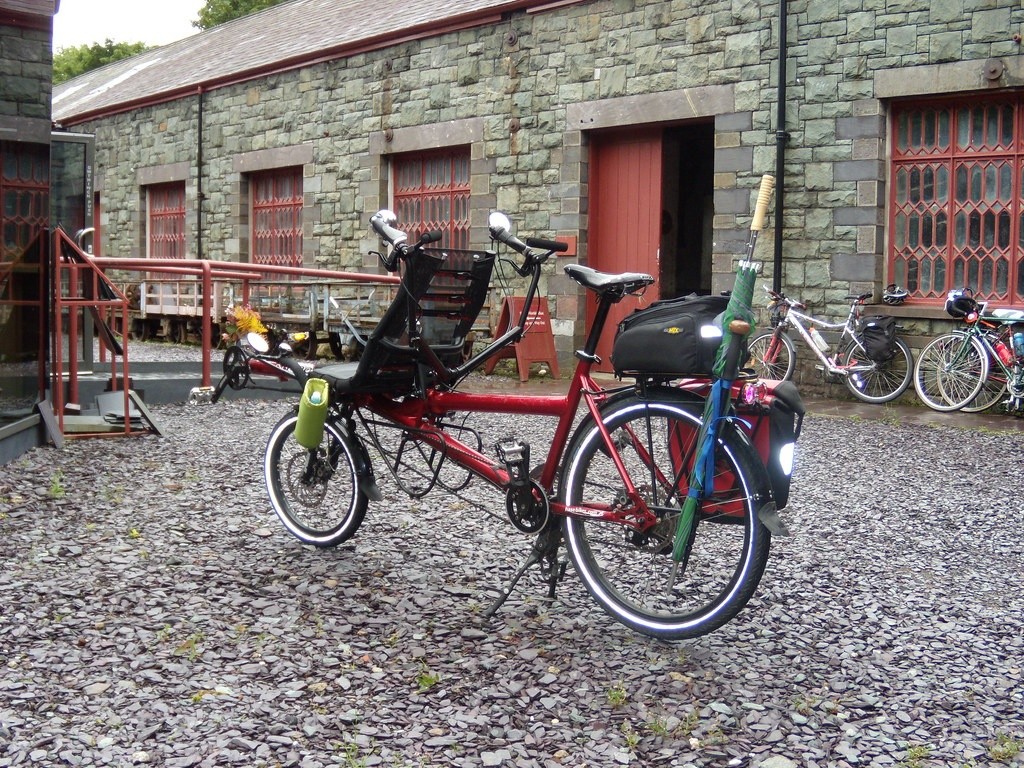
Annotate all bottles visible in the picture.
[311,392,322,404]
[1014,333,1024,356]
[993,340,1013,366]
[809,328,829,351]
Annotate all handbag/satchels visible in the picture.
[609,293,750,372]
[858,315,898,363]
[667,376,807,524]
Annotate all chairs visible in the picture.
[311,247,499,404]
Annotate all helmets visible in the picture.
[945,287,977,318]
[882,284,909,305]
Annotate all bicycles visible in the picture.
[213,208,792,642]
[910,286,1024,419]
[747,280,913,405]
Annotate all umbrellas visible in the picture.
[667,174,775,594]
